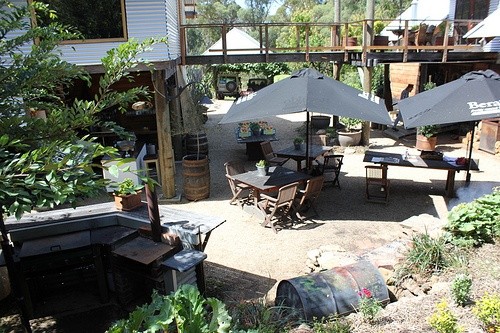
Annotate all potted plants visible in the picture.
[414,75,439,151]
[325,128,337,146]
[434,14,454,45]
[348,26,357,45]
[293,137,304,150]
[113,178,143,211]
[335,81,363,147]
[255,160,270,175]
[173,67,210,201]
[373,23,388,46]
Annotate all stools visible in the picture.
[142,154,161,184]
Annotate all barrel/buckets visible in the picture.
[182,154,210,201]
[186,131,208,156]
[275,260,390,326]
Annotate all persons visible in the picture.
[392,84,414,131]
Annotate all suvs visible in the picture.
[217,73,240,100]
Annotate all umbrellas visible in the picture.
[398,70,500,180]
[217,68,394,175]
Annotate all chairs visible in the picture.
[224,155,392,234]
[410,23,436,46]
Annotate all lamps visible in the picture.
[166,81,214,104]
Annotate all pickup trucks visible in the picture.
[247,78,268,93]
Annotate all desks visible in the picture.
[233,122,479,217]
[90,130,117,147]
[101,141,147,195]
[386,29,412,46]
